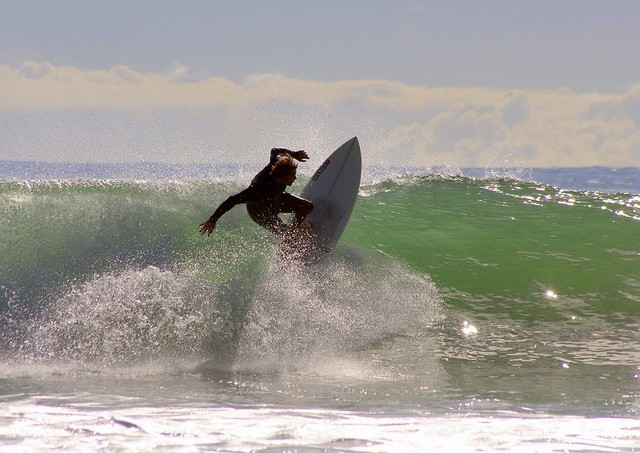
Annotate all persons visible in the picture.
[199,147,314,255]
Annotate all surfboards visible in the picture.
[273,136,362,266]
